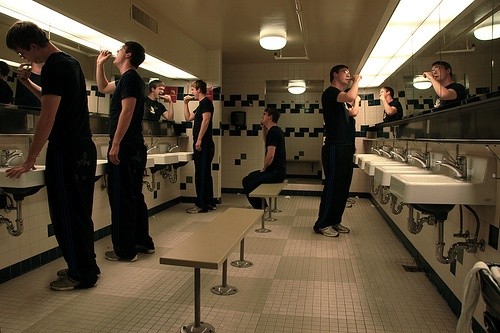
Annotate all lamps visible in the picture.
[287,85,306,96]
[413,80,432,90]
[473,24,499,41]
[258,34,286,52]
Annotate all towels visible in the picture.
[455,259,492,333]
[490,265,500,287]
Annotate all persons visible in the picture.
[0,61,13,105]
[313,65,362,237]
[184,79,217,213]
[141,80,174,134]
[5,20,100,290]
[423,61,464,112]
[343,88,361,117]
[96,41,155,262]
[241,107,286,210]
[379,86,403,122]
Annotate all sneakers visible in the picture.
[50,276,99,291]
[315,226,339,237]
[103,249,137,262]
[134,244,156,253]
[186,204,208,213]
[209,203,217,209]
[332,223,350,233]
[57,267,102,276]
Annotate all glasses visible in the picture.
[430,66,446,72]
[16,51,24,55]
[336,70,350,75]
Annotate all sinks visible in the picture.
[375,164,428,173]
[359,155,388,161]
[353,152,379,157]
[97,159,108,164]
[0,163,45,173]
[390,172,461,184]
[365,159,405,164]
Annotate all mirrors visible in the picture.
[1,14,189,137]
[366,1,500,144]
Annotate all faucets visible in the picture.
[370,145,382,155]
[146,137,160,152]
[378,147,394,157]
[167,142,178,150]
[389,148,408,162]
[1,148,24,165]
[435,157,463,178]
[407,151,430,167]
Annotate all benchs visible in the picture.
[160,207,265,332]
[249,179,286,233]
